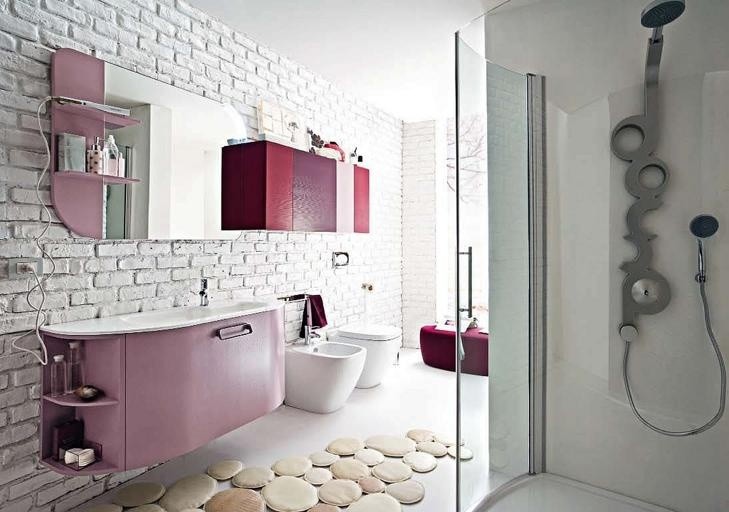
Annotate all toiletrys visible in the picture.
[117,153,125,179]
[86,136,108,174]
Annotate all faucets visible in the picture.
[303,326,320,344]
[199,278,208,304]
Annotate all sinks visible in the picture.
[121,307,215,326]
[209,301,269,314]
[286,341,369,414]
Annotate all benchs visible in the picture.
[420,325,488,377]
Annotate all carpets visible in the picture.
[93,429,475,511]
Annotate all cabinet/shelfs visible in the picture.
[39,332,125,476]
[222,139,369,233]
[49,48,142,242]
[126,306,285,469]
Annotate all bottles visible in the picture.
[50,354,67,398]
[63,341,83,395]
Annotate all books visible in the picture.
[436,315,473,334]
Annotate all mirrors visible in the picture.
[103,64,248,239]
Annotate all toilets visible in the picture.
[328,323,402,388]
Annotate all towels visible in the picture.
[299,295,328,338]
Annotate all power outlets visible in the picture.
[7,258,44,281]
